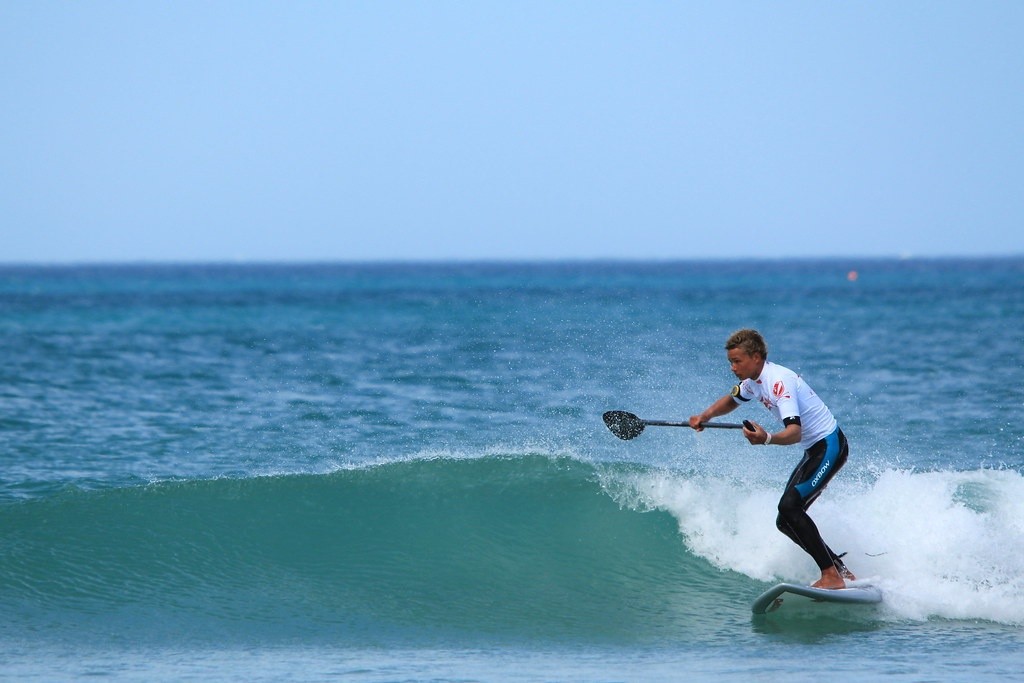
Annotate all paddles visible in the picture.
[602,410,756,441]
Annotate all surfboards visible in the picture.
[751,582,884,617]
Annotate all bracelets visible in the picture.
[763,433,771,445]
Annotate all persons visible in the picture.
[690,330,856,589]
